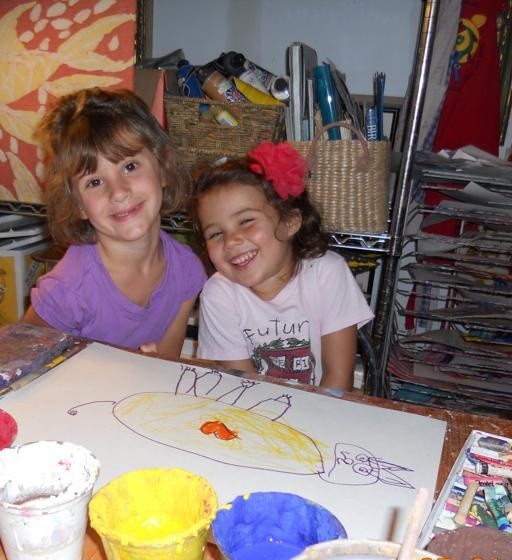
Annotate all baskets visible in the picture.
[163,93,285,218]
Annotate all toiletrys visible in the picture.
[175,52,289,126]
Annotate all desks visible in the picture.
[2,320,512,560]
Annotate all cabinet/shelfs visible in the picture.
[0,141,512,423]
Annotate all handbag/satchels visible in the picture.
[286,122,392,234]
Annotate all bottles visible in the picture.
[197,50,291,127]
[175,59,209,115]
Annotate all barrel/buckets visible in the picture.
[211,490,348,560]
[289,538,446,560]
[87,466,214,560]
[0,440,100,560]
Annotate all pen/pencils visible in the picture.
[443,437,512,532]
[387,508,401,541]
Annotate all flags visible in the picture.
[387,2,505,334]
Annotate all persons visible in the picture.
[186,141,377,395]
[20,83,209,359]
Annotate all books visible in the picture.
[379,144,510,422]
[277,42,387,146]
